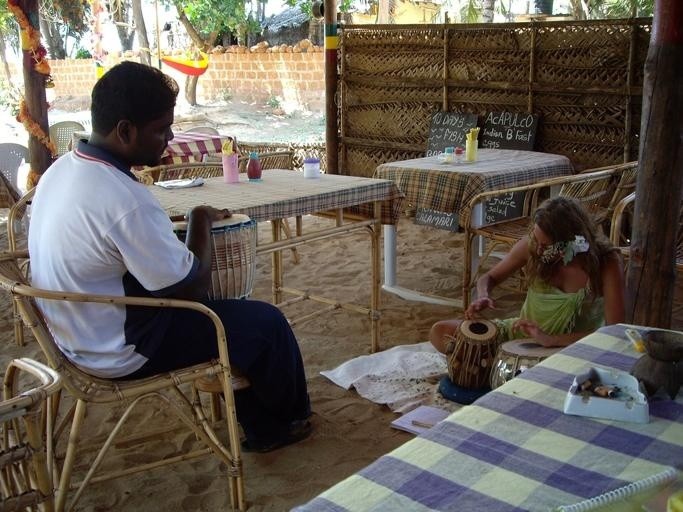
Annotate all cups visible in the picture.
[222,153,238,183]
[466,139,478,162]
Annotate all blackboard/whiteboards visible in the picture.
[413,111,540,232]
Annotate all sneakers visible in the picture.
[255,421,313,454]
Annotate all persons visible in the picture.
[429,196,626,355]
[27,61,314,453]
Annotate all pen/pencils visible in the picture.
[412,421,433,428]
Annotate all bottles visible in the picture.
[444,146,453,163]
[247,152,262,182]
[303,158,321,179]
[455,146,464,165]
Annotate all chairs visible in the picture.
[1,357,64,511]
[462,160,682,310]
[0,120,300,346]
[1,252,246,510]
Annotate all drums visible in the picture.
[172,213,257,393]
[445,318,501,389]
[488,337,565,390]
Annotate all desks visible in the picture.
[288,323,683,511]
[143,145,575,354]
